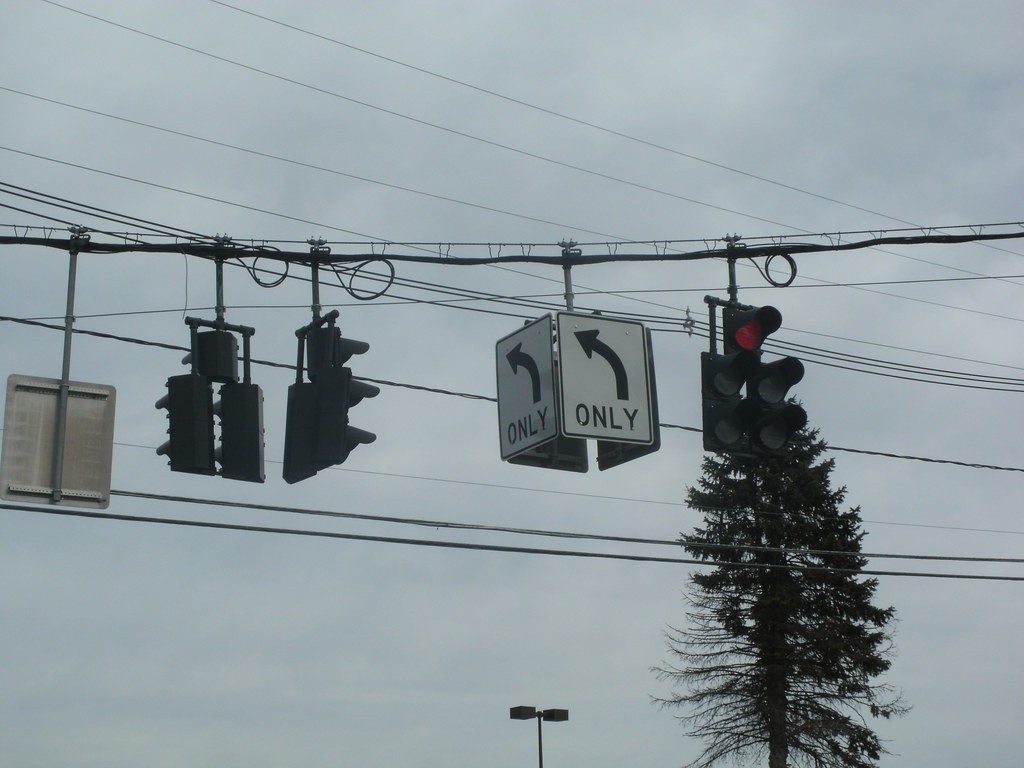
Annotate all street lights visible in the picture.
[510,706,569,768]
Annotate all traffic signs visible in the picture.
[555,309,656,446]
[495,312,559,462]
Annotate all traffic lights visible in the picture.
[306,327,370,383]
[213,383,268,483]
[742,356,809,457]
[700,350,762,453]
[722,306,781,381]
[313,367,379,467]
[182,330,238,383]
[153,374,216,476]
